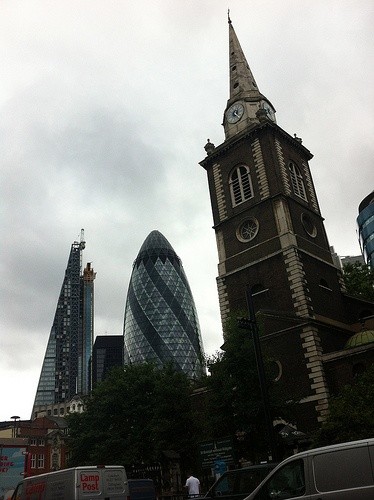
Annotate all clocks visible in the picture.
[263,102,275,122]
[226,103,245,123]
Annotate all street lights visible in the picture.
[11,416,20,437]
[236,282,279,462]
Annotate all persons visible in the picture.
[185,472,200,498]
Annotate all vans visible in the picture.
[243,438,374,500]
[10,465,155,500]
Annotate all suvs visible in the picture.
[204,464,303,500]
